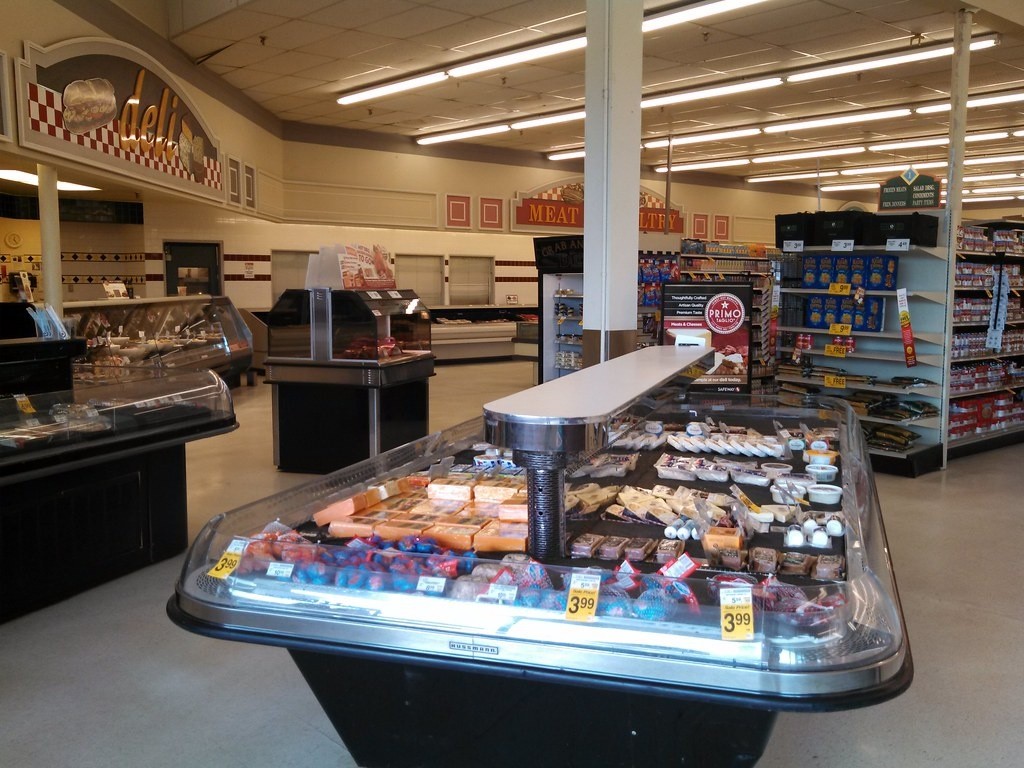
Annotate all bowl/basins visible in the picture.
[93,331,222,360]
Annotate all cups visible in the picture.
[177,286,187,296]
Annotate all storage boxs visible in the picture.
[775,212,1024,441]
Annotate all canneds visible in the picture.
[795,333,856,353]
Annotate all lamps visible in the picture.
[335,0,1024,204]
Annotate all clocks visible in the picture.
[5,232,23,248]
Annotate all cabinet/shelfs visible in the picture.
[775,246,949,479]
[680,253,772,406]
[947,251,1024,461]
[554,293,582,370]
